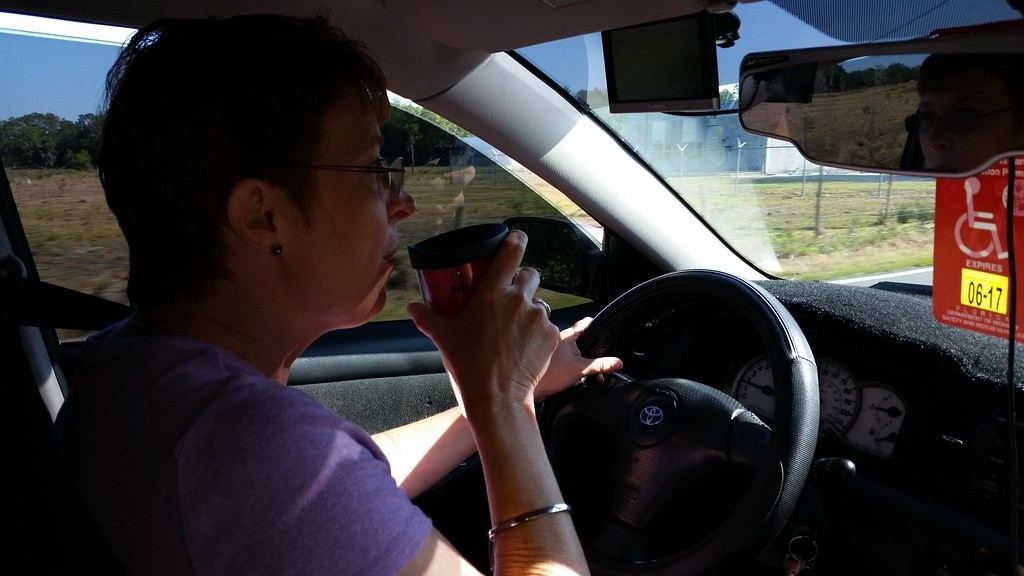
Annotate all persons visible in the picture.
[51,8,624,576]
[915,51,1023,173]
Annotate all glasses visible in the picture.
[248,157,406,201]
[905,106,1016,135]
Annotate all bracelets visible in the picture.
[489,503,573,542]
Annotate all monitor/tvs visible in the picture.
[601,11,720,113]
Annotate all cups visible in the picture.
[408,222,510,319]
